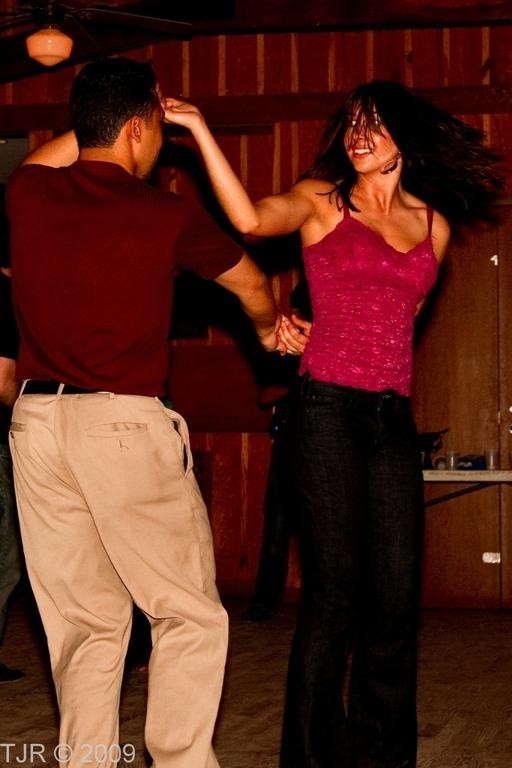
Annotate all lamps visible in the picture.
[22,3,77,66]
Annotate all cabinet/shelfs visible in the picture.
[421,484,512,610]
[410,206,511,468]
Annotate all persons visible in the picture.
[161,80,512,768]
[242,284,310,623]
[1,55,300,768]
[1,220,25,679]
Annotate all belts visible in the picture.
[23,379,99,393]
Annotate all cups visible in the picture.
[429,453,446,470]
[484,448,499,470]
[444,449,459,471]
